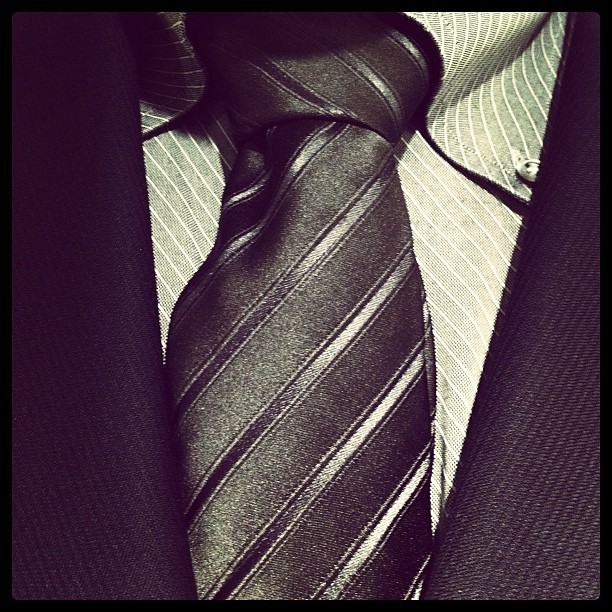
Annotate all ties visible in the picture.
[165,12,437,601]
